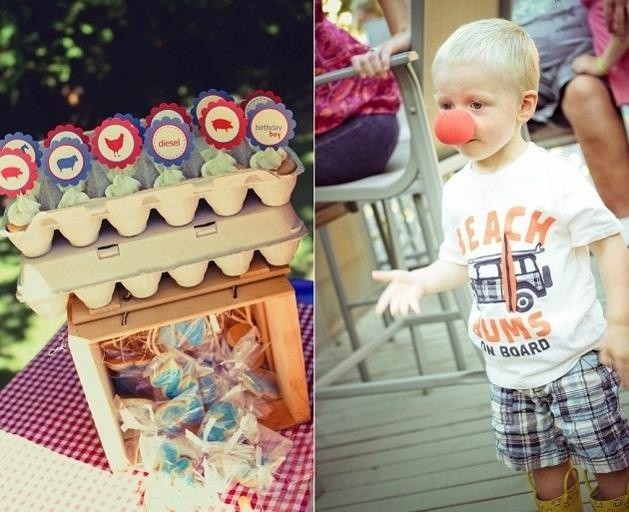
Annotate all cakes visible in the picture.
[0,137,295,232]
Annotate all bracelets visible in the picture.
[596,56,608,75]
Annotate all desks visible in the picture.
[0,300,314,511]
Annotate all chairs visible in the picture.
[315,0,515,397]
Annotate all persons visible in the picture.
[370,19,629,512]
[316,0,401,188]
[509,0,629,246]
[572,0,629,144]
[350,0,411,78]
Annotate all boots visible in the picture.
[528,467,581,512]
[584,466,628,511]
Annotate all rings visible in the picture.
[370,48,378,51]
